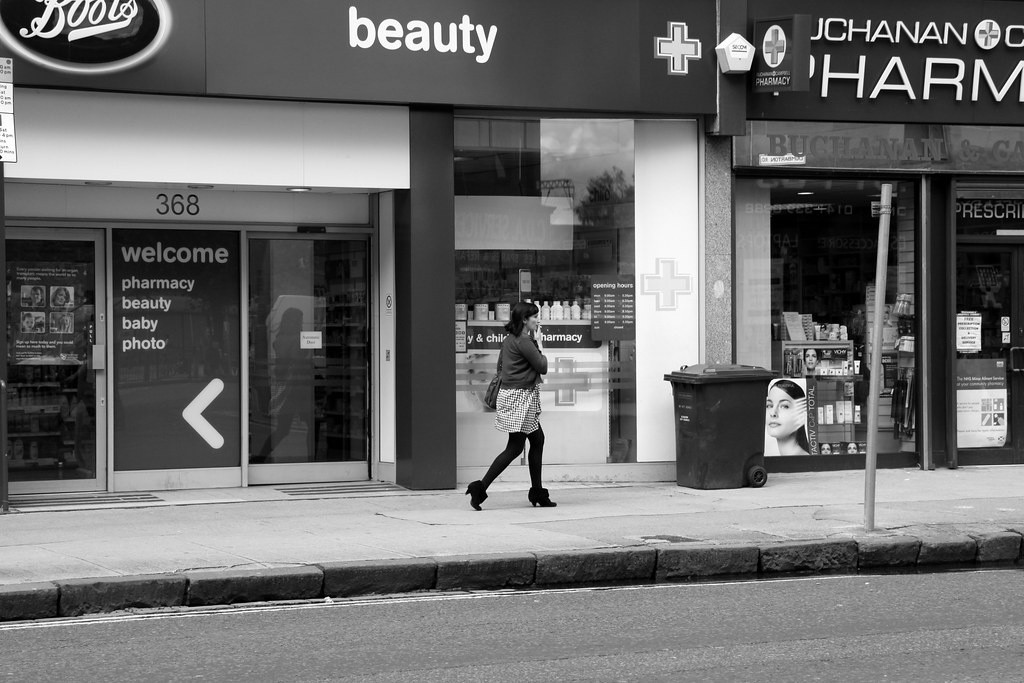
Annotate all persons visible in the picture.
[52,288,71,306]
[766,381,809,456]
[258,308,326,462]
[22,313,36,332]
[803,348,822,375]
[27,286,45,306]
[847,443,857,454]
[821,444,831,454]
[464,302,557,512]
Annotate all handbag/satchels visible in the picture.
[485,376,502,409]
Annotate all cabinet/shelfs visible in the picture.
[250,282,365,451]
[5,361,81,470]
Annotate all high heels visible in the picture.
[465,480,487,510]
[528,487,557,507]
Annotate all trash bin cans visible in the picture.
[664,364,773,490]
